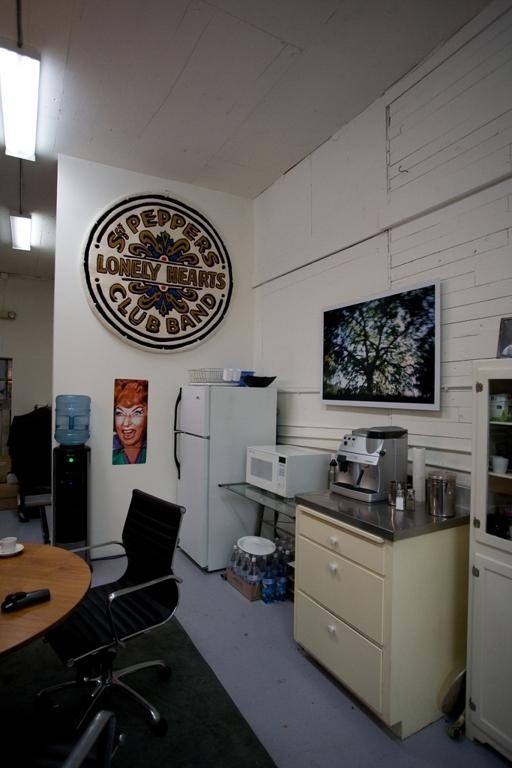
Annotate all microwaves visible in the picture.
[246,444,326,500]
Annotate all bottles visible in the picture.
[425,470,457,519]
[388,474,416,512]
[227,532,296,602]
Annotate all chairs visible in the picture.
[0,689,127,768]
[32,489,186,739]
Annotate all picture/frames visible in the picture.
[320,281,440,412]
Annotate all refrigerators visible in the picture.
[173,381,277,574]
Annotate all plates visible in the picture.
[2,545,28,559]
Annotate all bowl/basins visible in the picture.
[238,370,276,387]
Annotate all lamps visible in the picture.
[0,0,41,252]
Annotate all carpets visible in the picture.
[0,615,278,768]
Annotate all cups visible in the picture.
[490,455,508,473]
[2,537,17,550]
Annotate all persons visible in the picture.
[112,379,148,464]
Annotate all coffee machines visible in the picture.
[328,425,407,505]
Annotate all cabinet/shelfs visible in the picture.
[294,495,468,741]
[466,358,512,762]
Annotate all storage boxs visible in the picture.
[225,567,263,601]
[0,456,19,511]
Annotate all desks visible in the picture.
[218,483,296,537]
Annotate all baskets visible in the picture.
[185,367,224,383]
